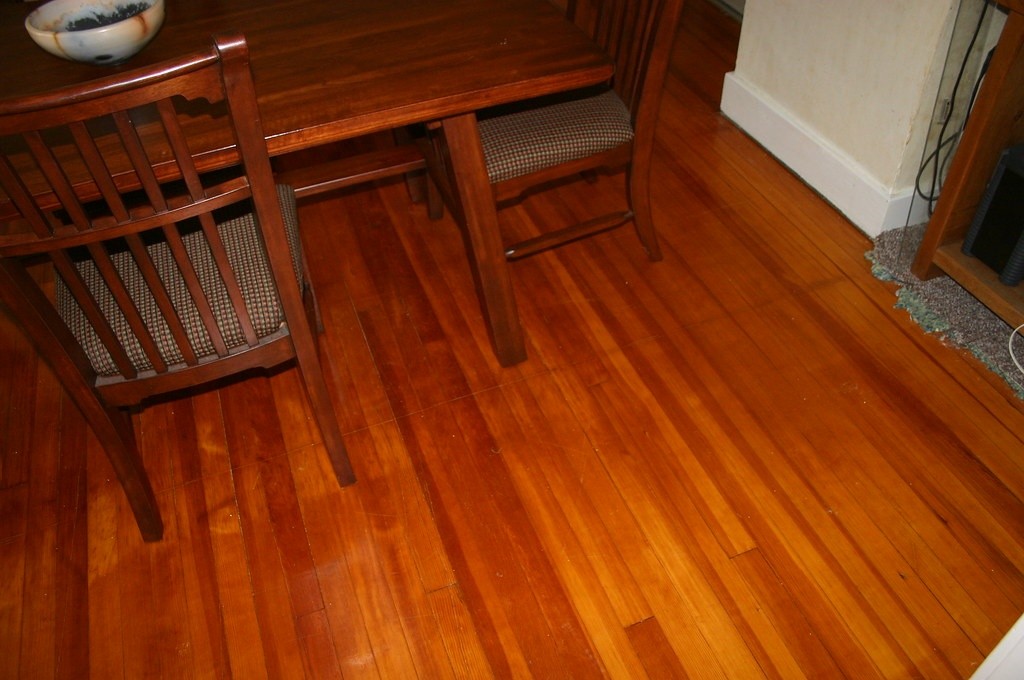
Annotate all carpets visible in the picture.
[863,223,1024,405]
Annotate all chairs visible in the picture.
[426,0,681,262]
[0,31,356,543]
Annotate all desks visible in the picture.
[0,0,617,371]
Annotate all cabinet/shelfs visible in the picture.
[911,0,1024,332]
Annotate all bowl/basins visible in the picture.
[24,0,165,64]
